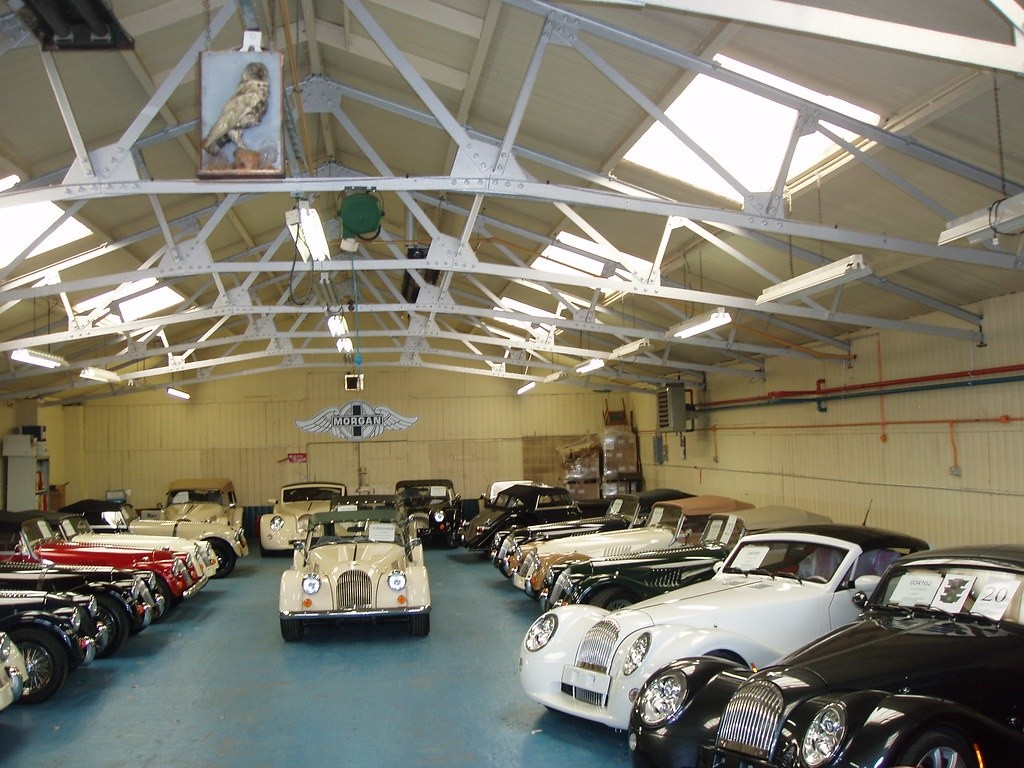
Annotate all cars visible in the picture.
[490,488,697,578]
[0,631,30,712]
[0,583,109,705]
[58,499,249,579]
[628,546,1024,768]
[456,484,609,551]
[18,510,219,577]
[279,510,431,641]
[1,510,209,616]
[157,478,244,527]
[503,495,758,596]
[0,529,165,659]
[259,482,348,556]
[395,480,463,549]
[518,525,929,730]
[538,505,832,615]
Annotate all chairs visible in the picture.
[796,547,901,585]
[601,397,628,427]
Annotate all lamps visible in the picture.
[12,197,874,400]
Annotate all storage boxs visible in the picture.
[563,429,638,502]
